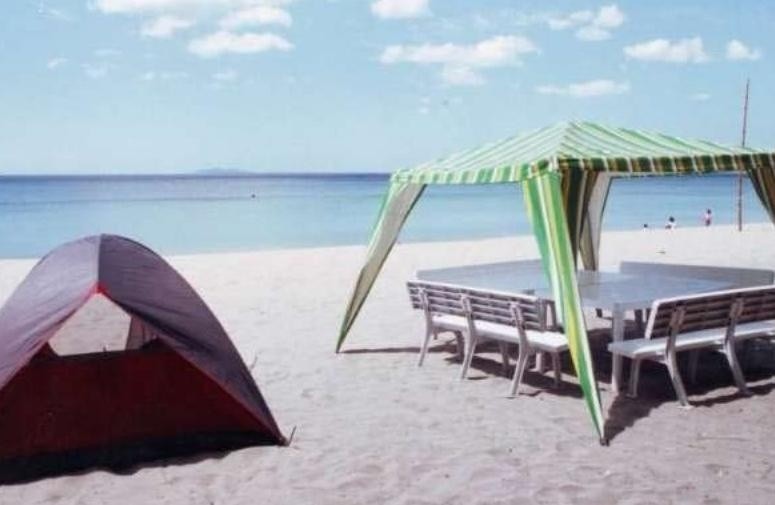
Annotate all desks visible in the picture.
[440,270,732,397]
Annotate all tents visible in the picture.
[0,233,292,477]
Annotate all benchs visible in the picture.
[405,280,568,400]
[416,258,541,279]
[594,261,775,323]
[608,284,775,406]
